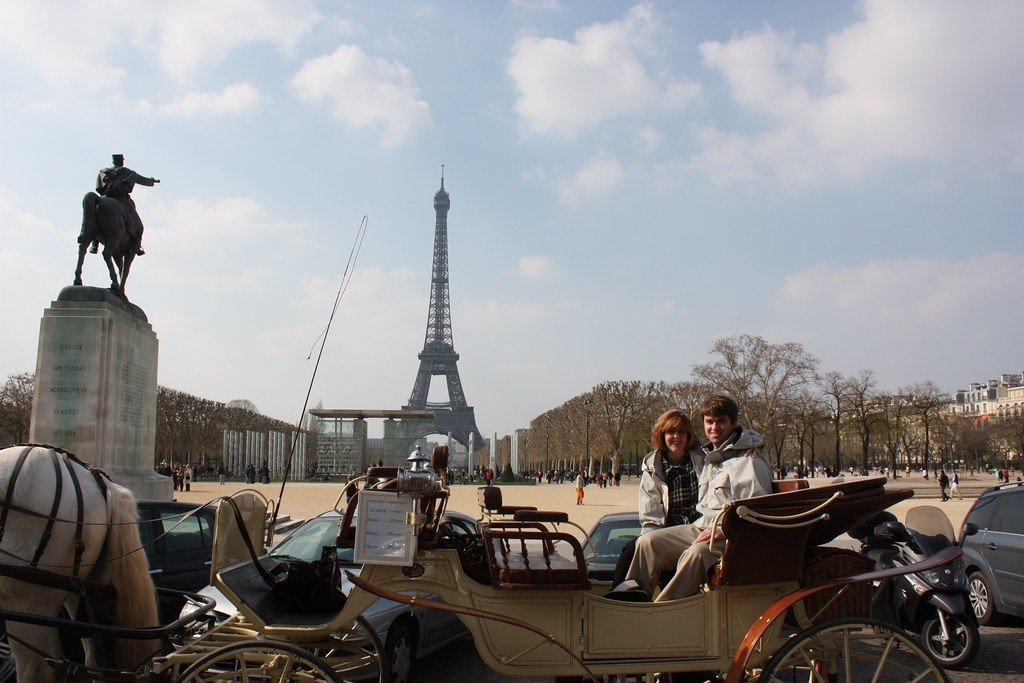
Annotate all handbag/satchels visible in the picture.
[186,475,190,479]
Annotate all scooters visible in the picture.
[847,505,980,671]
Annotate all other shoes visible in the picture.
[940,500,945,502]
[576,502,579,505]
[580,502,584,505]
[957,497,963,500]
[946,496,950,500]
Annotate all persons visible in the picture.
[776,465,1021,502]
[447,467,621,505]
[155,461,270,492]
[345,460,383,504]
[89,154,160,256]
[608,410,707,592]
[626,394,772,603]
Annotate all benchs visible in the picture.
[706,480,810,577]
[336,478,448,551]
[165,532,210,551]
[474,486,591,591]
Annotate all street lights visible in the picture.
[583,398,592,467]
[545,419,550,473]
[523,430,528,471]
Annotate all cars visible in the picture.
[569,512,650,572]
[126,493,218,648]
[175,509,504,683]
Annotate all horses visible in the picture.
[74,192,136,302]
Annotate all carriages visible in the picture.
[1,429,965,683]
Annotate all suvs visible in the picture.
[955,480,1024,628]
